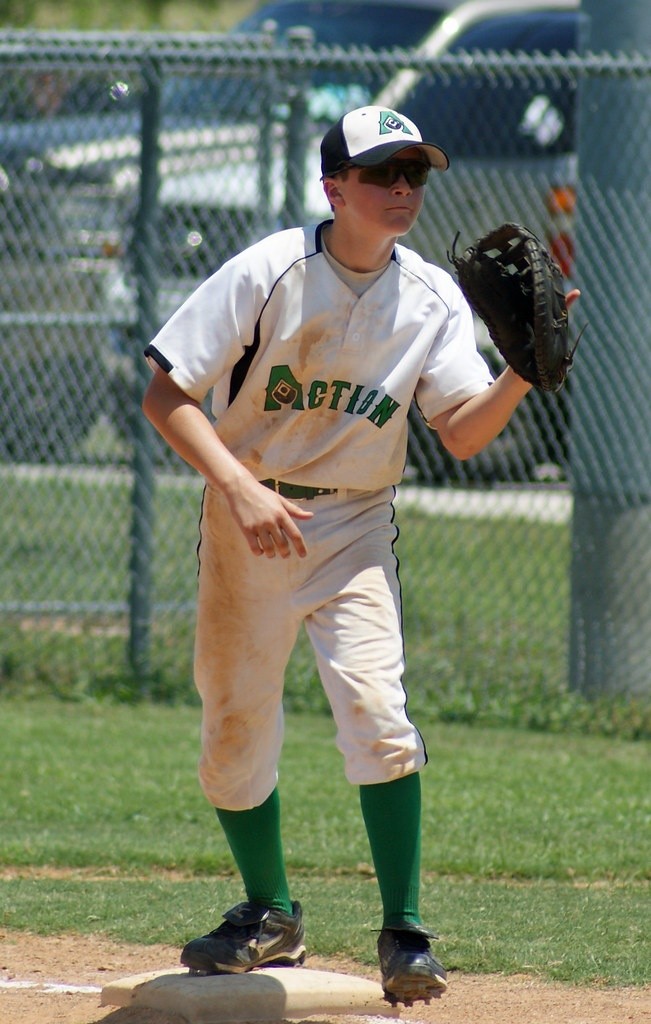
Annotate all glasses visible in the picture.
[351,158,430,189]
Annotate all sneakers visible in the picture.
[375,923,445,1006]
[180,898,307,975]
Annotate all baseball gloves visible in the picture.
[453,216,577,397]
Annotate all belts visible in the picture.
[263,476,336,501]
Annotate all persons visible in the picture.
[141,103,582,1005]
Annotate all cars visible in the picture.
[0,1,587,484]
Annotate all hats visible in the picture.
[320,106,450,180]
